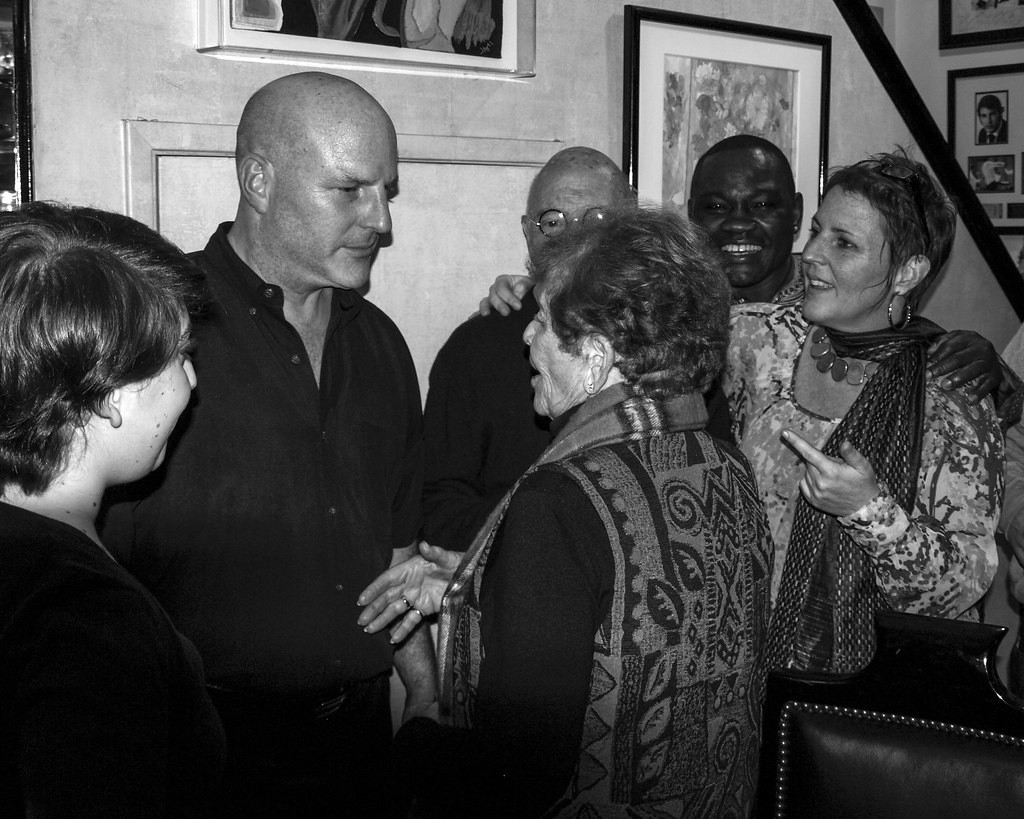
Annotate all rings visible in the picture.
[411,607,424,620]
[402,595,412,610]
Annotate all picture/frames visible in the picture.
[947,64,1023,235]
[936,0,1024,50]
[622,5,833,255]
[194,0,538,80]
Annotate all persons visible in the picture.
[466,143,1024,674]
[981,157,1008,191]
[687,134,1024,431]
[95,70,424,819]
[358,197,776,819]
[994,243,1024,697]
[0,200,244,819]
[977,94,1008,144]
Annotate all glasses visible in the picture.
[527,207,607,238]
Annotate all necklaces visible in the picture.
[811,325,881,385]
